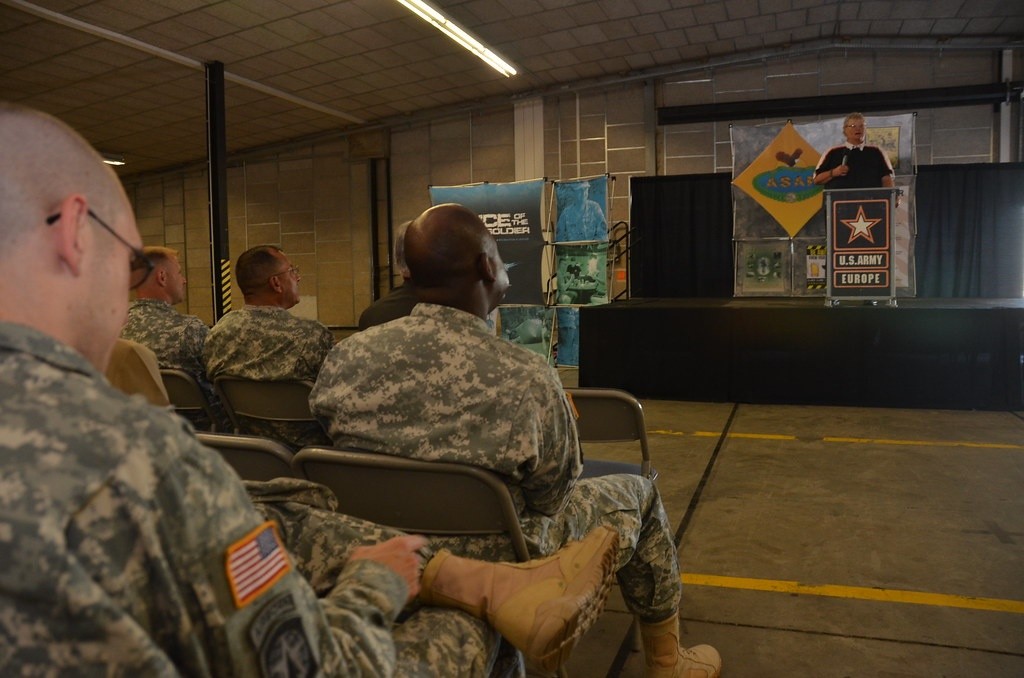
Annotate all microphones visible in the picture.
[842,148,852,166]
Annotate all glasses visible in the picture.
[846,124,863,129]
[272,265,301,280]
[46,209,155,290]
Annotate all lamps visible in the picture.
[397,0,519,77]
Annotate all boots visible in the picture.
[639,610,721,678]
[419,525,620,673]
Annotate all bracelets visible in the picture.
[830,168,835,179]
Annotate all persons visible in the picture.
[813,113,901,306]
[203,246,335,453]
[556,182,608,240]
[0,103,622,678]
[557,314,578,366]
[557,258,592,304]
[307,202,722,678]
[359,220,416,333]
[121,246,210,432]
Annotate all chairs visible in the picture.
[163,370,658,678]
